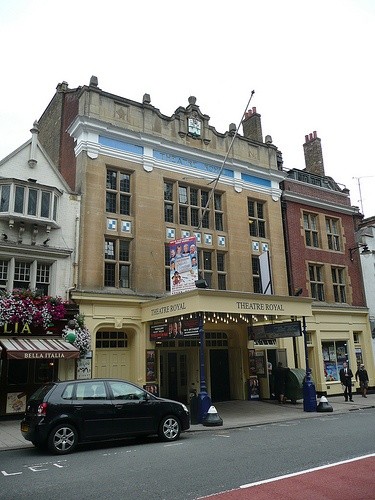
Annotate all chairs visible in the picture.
[84,387,104,398]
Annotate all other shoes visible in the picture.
[362,395,367,398]
[345,399,354,402]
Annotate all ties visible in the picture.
[345,369,346,373]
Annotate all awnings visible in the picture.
[0,337,80,358]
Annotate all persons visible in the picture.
[170,244,198,284]
[340,363,355,402]
[356,363,369,398]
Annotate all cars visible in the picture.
[20,377,192,455]
